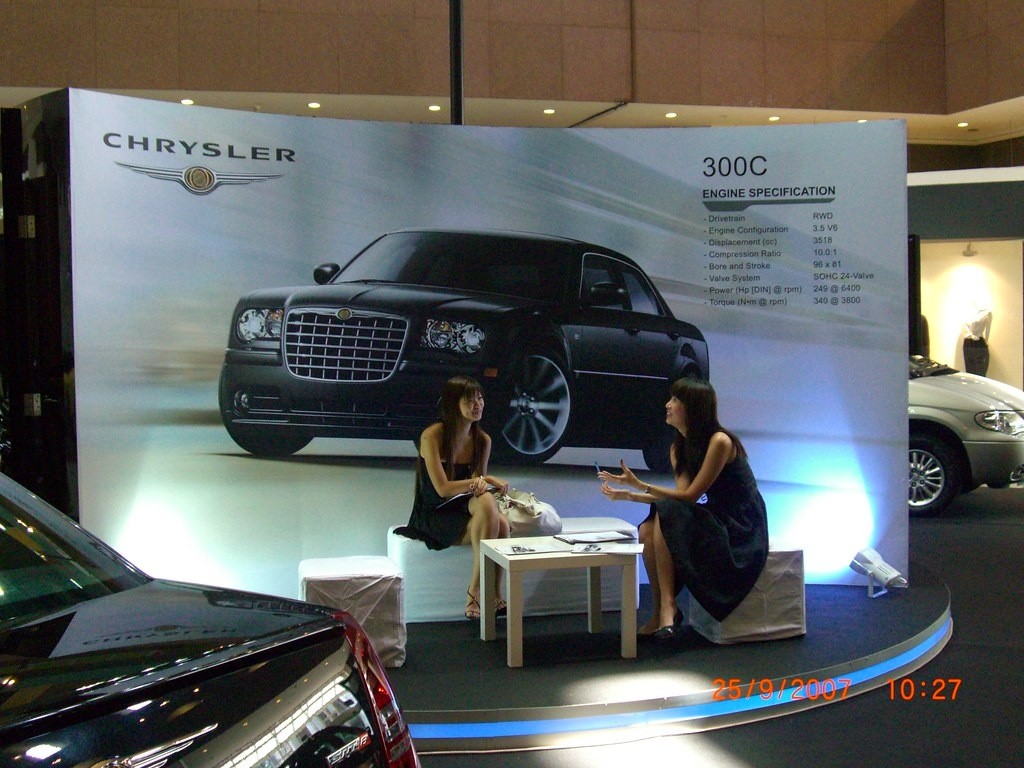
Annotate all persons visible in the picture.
[393,376,510,630]
[962,304,992,376]
[597,377,768,640]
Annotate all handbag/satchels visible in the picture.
[494,488,563,539]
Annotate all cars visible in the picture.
[220,225,714,481]
[907,352,1024,518]
[0,468,420,768]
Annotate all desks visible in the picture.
[479,537,639,667]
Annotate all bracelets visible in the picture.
[480,476,482,479]
[645,485,650,493]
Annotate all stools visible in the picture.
[298,555,407,669]
[688,548,807,646]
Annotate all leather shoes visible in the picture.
[636,627,658,642]
[654,607,684,640]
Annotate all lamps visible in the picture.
[849,547,909,598]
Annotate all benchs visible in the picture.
[387,516,639,623]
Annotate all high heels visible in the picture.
[465,586,480,620]
[494,598,507,615]
